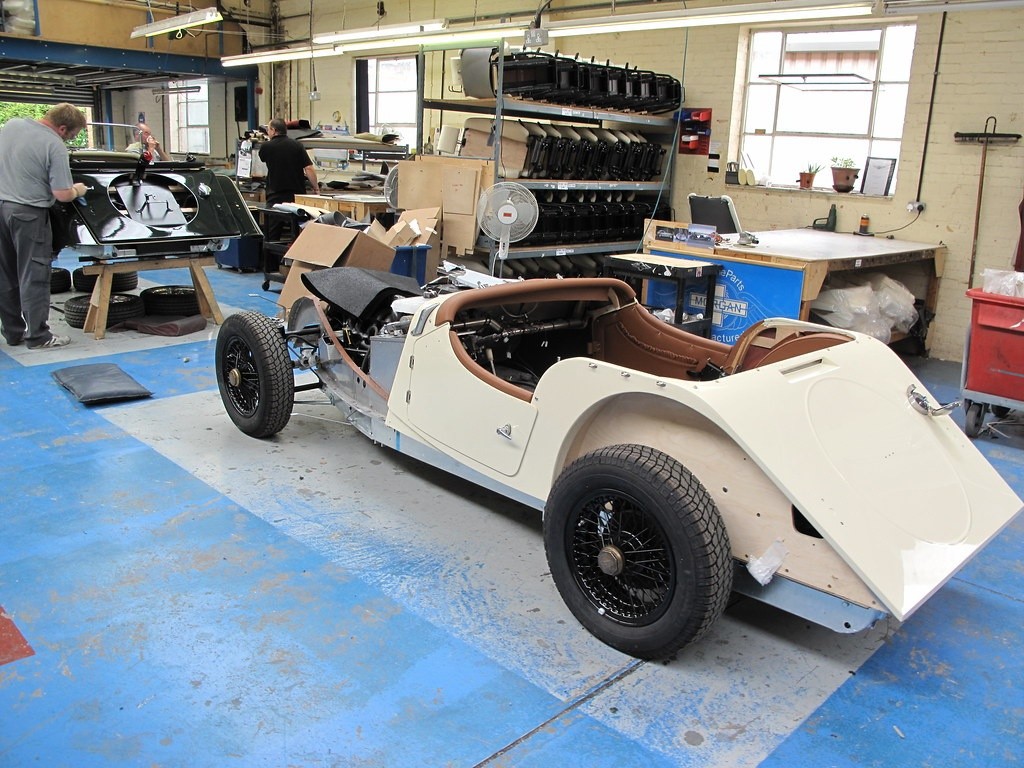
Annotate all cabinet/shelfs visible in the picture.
[606,254,722,338]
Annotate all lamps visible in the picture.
[152,79,201,94]
[130,0,559,67]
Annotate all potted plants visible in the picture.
[800,162,825,189]
[830,157,860,192]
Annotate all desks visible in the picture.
[644,225,947,358]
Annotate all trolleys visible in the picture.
[956,286,1024,437]
[257,207,310,291]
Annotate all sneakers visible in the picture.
[19,330,30,341]
[28,334,72,349]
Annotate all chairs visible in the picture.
[687,193,742,234]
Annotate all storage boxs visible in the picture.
[237,149,268,179]
[276,208,441,311]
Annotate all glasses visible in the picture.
[59,124,77,140]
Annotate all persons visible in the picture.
[127,122,173,160]
[258,118,320,271]
[0,102,85,350]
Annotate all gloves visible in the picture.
[73,182,87,197]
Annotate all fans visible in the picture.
[475,182,539,278]
[384,164,398,209]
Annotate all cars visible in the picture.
[51,122,265,263]
[214,258,1024,661]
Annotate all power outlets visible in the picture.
[908,202,926,214]
